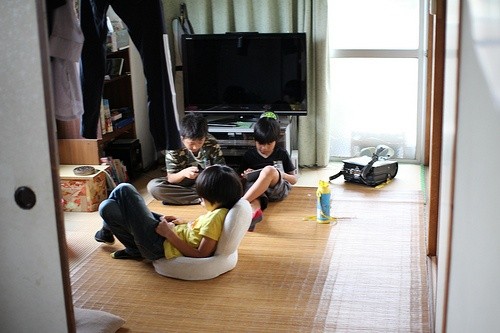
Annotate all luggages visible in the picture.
[330,149,398,187]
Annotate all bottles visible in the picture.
[316,180,330,224]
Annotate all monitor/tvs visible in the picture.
[181,34,308,124]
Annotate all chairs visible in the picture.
[153,199,254,279]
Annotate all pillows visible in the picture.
[74,307,127,333]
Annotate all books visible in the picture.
[96,97,122,141]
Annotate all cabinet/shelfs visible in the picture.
[57,34,158,176]
[207,116,298,169]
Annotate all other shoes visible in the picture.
[95,229,114,245]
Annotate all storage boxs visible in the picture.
[56,139,104,165]
[57,162,111,213]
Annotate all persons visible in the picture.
[94,163,244,261]
[146,112,227,205]
[238,117,297,232]
